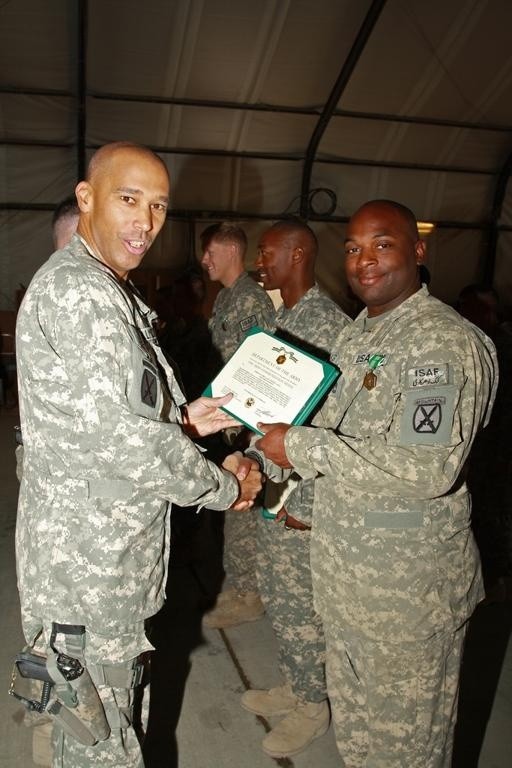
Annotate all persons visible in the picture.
[11,136,267,763]
[48,193,90,251]
[218,199,495,766]
[190,221,280,629]
[236,215,359,763]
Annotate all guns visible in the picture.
[16,652,83,684]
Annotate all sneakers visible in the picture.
[201,583,265,627]
[238,686,292,716]
[262,700,331,759]
